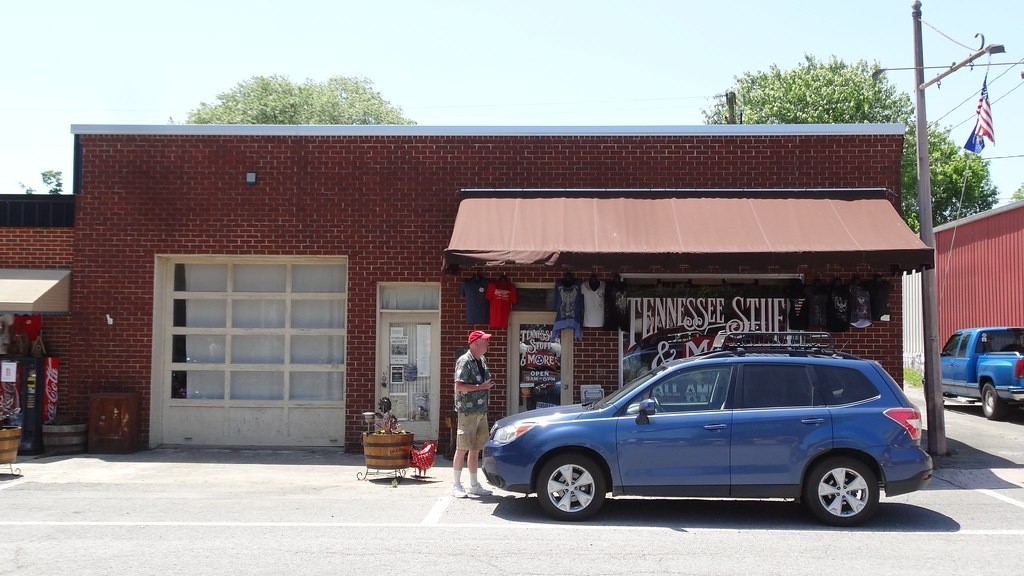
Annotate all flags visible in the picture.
[964,73,997,154]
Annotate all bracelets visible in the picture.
[476,384,479,391]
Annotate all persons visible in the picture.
[451,330,494,499]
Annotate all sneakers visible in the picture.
[468,483,493,496]
[450,484,468,498]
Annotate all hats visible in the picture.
[468,330,492,346]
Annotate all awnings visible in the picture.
[443,188,936,277]
[0,267,71,317]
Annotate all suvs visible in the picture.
[482,332,934,528]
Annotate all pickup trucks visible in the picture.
[940,326,1024,421]
